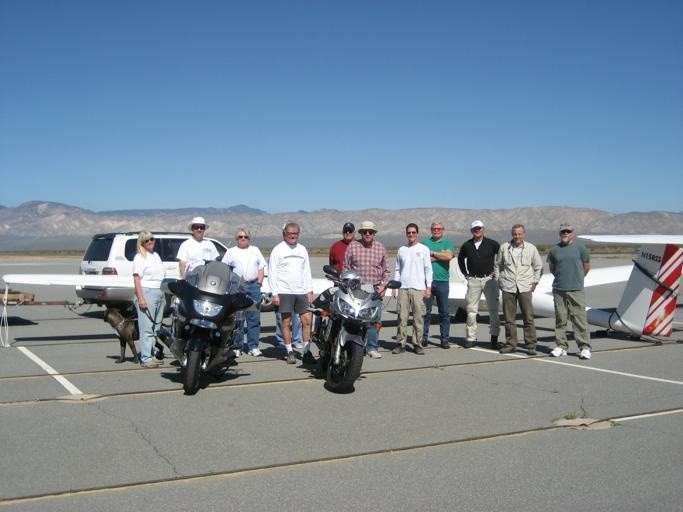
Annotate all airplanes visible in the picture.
[381,233,683,345]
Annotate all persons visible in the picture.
[419,220,456,349]
[132,230,166,369]
[492,223,542,354]
[457,220,500,349]
[268,222,318,364]
[220,228,265,357]
[273,298,302,361]
[176,216,220,278]
[545,222,592,360]
[392,223,433,354]
[341,221,390,359]
[328,222,355,287]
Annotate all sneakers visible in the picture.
[579,348,592,360]
[247,347,263,357]
[490,335,518,354]
[231,348,243,358]
[549,345,568,358]
[302,350,319,365]
[440,340,450,350]
[140,356,165,369]
[365,349,383,360]
[291,339,304,350]
[286,349,297,364]
[463,340,479,348]
[526,347,537,356]
[412,338,429,355]
[391,342,406,354]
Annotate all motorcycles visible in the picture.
[308,263,403,398]
[156,257,254,398]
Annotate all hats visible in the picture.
[357,220,378,235]
[470,220,485,231]
[342,222,356,231]
[187,216,211,231]
[559,222,574,233]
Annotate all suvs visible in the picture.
[74,228,274,318]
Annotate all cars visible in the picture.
[0,287,36,305]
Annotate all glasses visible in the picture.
[362,231,375,235]
[238,235,250,239]
[142,237,155,244]
[432,227,444,231]
[407,231,417,234]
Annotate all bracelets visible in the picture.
[256,282,262,286]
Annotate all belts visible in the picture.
[468,269,495,278]
[243,277,258,285]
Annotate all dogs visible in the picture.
[103,308,172,365]
